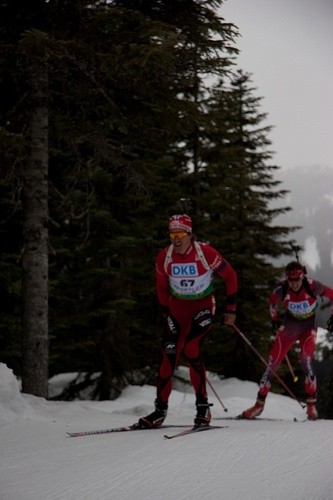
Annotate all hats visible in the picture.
[168,213,193,233]
[285,263,303,278]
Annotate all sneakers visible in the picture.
[194,399,214,424]
[306,398,318,419]
[139,399,168,427]
[242,399,265,418]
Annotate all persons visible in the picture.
[133,214,237,429]
[237,261,333,421]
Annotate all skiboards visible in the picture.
[66,424,230,439]
[212,416,307,423]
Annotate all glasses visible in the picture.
[169,231,188,239]
[287,273,303,282]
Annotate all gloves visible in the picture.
[327,315,333,332]
[272,320,280,336]
